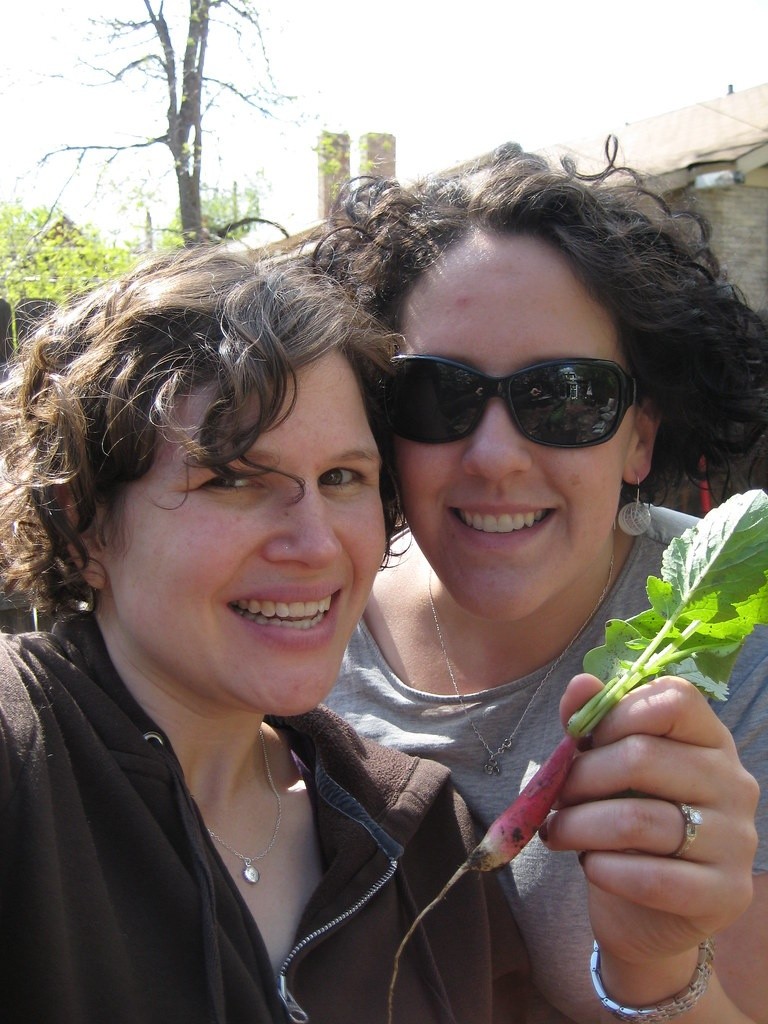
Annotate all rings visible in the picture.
[668,799,704,858]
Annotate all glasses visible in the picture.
[369,353,643,448]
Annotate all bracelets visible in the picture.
[590,935,716,1024]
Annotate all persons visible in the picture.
[0,144,768,1024]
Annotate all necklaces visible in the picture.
[429,554,614,776]
[208,729,281,883]
